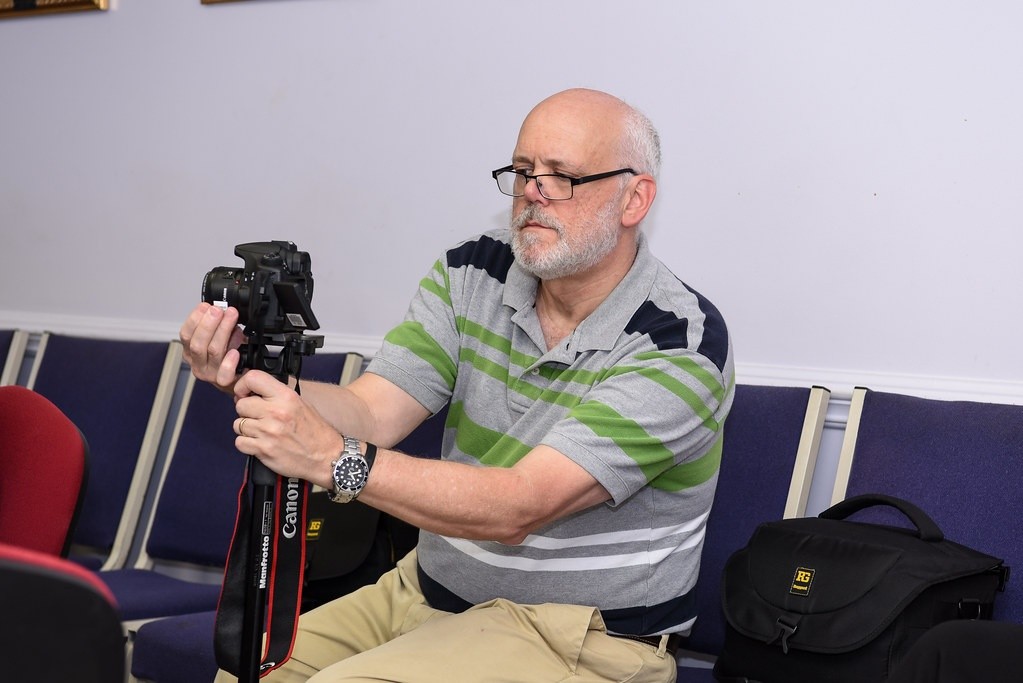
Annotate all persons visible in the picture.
[181,86,737,683]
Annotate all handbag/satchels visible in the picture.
[710,492,1009,683]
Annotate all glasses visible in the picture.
[492,165,639,200]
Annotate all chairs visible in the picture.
[0,328,1023,683]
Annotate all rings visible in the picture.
[239,417,248,434]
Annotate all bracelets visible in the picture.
[363,439,379,472]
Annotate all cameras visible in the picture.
[202,240,321,332]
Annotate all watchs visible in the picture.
[328,432,370,503]
